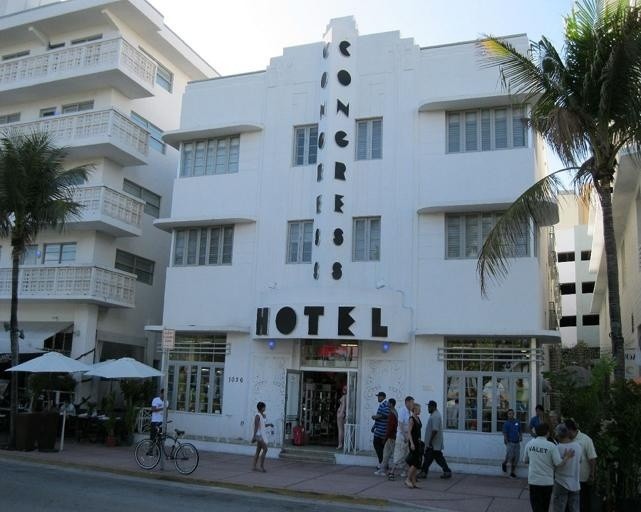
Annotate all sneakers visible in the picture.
[374,470,386,476]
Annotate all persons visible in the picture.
[404,403,422,489]
[526,405,547,438]
[250,402,273,472]
[388,396,415,481]
[502,409,522,478]
[553,424,584,512]
[565,418,597,512]
[61,391,96,416]
[446,385,478,430]
[146,389,169,456]
[484,377,504,430]
[515,378,527,411]
[1,390,30,412]
[524,422,576,512]
[374,398,400,475]
[417,400,451,479]
[371,392,390,469]
[336,386,346,449]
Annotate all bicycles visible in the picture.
[132,421,200,475]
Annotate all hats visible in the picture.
[426,401,436,405]
[376,392,385,396]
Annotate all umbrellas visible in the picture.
[5,351,90,389]
[83,357,166,406]
